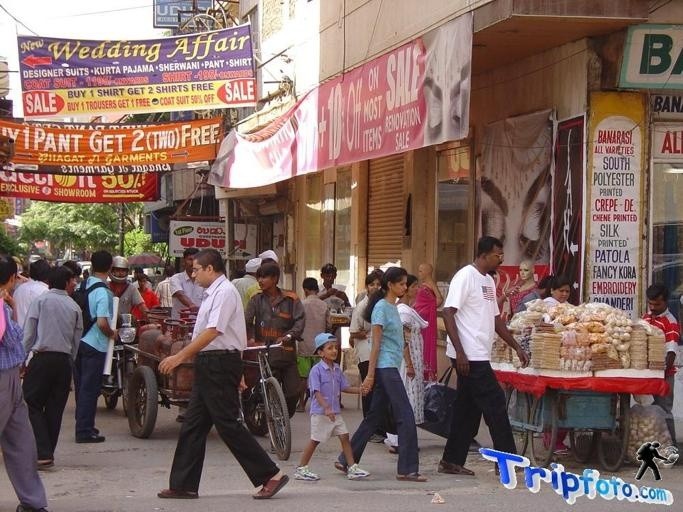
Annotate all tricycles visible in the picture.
[123,339,291,460]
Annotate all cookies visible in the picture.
[490,322,563,370]
[630,328,665,370]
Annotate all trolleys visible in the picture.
[505,385,631,472]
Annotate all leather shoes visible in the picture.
[76,433,105,443]
[438,460,475,475]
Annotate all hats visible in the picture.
[312,332,337,355]
[245,257,263,272]
[259,250,279,263]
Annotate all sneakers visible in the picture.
[368,433,384,443]
[346,463,371,480]
[294,464,320,481]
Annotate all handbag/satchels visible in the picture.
[416,367,457,439]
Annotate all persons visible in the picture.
[348,273,390,442]
[294,331,370,481]
[155,247,290,500]
[315,264,352,408]
[11,259,53,380]
[154,265,176,317]
[510,258,540,312]
[242,256,284,328]
[639,281,681,464]
[168,248,219,421]
[384,272,427,455]
[0,252,184,327]
[334,265,429,483]
[476,119,554,267]
[69,250,118,444]
[293,276,333,411]
[19,265,85,470]
[243,261,307,453]
[437,235,530,477]
[424,14,470,144]
[534,275,576,452]
[354,268,387,304]
[411,262,444,382]
[0,253,50,512]
[512,274,556,312]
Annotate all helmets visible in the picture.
[108,256,130,283]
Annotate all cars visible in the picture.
[74,260,98,289]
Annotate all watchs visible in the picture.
[285,334,292,340]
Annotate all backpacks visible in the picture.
[69,277,110,339]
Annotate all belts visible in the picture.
[197,350,238,357]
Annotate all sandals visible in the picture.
[396,473,427,481]
[335,462,347,473]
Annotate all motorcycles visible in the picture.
[100,313,150,415]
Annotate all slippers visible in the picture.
[158,489,198,499]
[252,475,289,499]
[36,462,54,469]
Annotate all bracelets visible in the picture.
[364,375,374,381]
[406,366,412,369]
